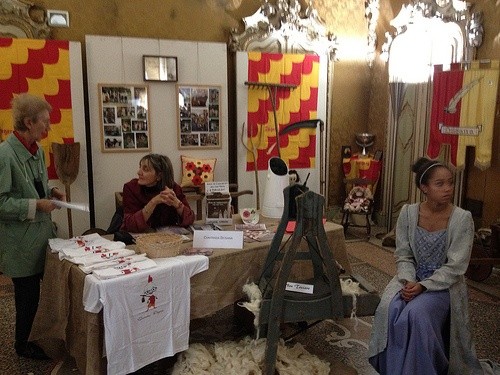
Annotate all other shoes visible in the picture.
[14,341,49,360]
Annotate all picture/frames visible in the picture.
[97,82,152,152]
[142,54,179,82]
[175,84,222,149]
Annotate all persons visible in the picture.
[119,153,195,233]
[367,156,485,375]
[1,94,67,359]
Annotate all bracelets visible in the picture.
[144,206,152,214]
[176,202,183,209]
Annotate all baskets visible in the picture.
[135,233,184,258]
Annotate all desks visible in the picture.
[49,210,345,375]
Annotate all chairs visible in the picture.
[339,198,374,238]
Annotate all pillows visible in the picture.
[180,155,217,189]
